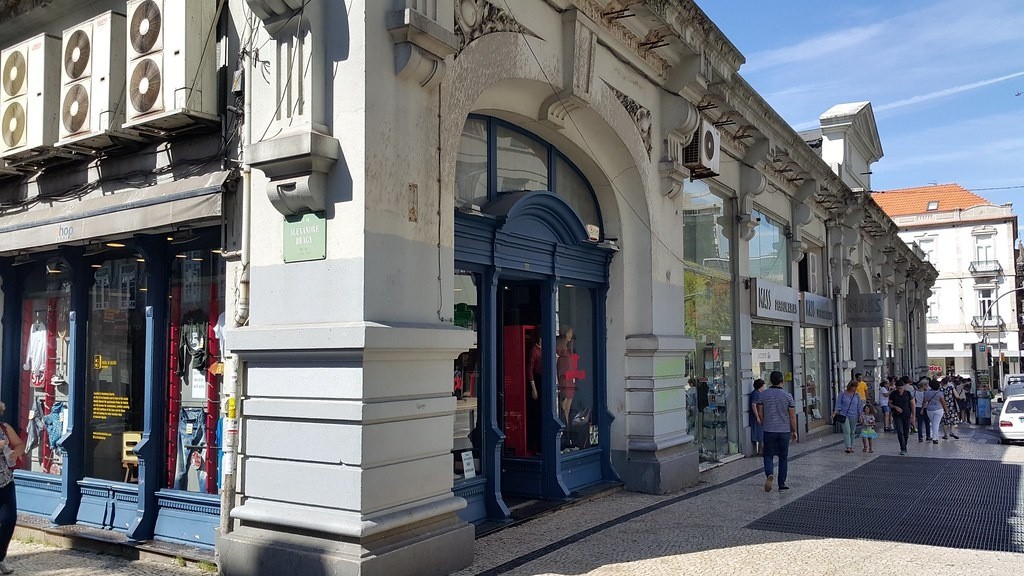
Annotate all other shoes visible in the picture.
[1,561,14,573]
[950,433,959,439]
[926,437,932,440]
[571,447,579,451]
[914,427,918,432]
[919,438,923,442]
[850,448,854,452]
[933,440,938,443]
[779,487,790,491]
[869,451,873,453]
[942,436,948,439]
[560,451,564,454]
[900,451,907,454]
[863,446,867,452]
[564,448,570,452]
[845,450,850,453]
[884,426,890,431]
[765,475,775,492]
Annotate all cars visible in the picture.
[997,394,1024,445]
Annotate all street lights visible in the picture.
[988,275,1004,392]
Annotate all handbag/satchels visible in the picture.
[0,423,23,470]
[954,399,960,412]
[834,414,846,423]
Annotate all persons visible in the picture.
[748,372,976,492]
[685,379,697,435]
[527,323,580,455]
[0,402,24,574]
